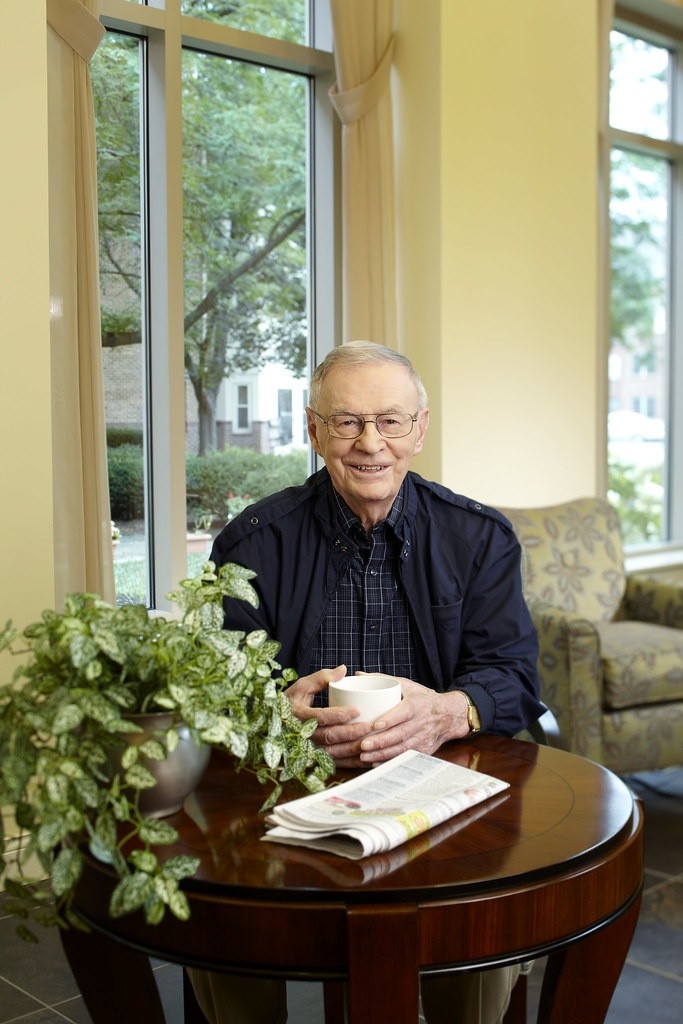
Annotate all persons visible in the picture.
[334,744,481,771]
[183,340,548,1024]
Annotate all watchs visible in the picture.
[458,689,480,738]
[464,746,480,770]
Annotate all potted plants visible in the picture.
[0,562,335,944]
[187,515,212,553]
[110,521,121,557]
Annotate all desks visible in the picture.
[56,736,646,1024]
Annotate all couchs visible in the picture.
[488,497,683,773]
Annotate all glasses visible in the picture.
[310,411,420,440]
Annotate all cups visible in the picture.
[329,671,404,771]
